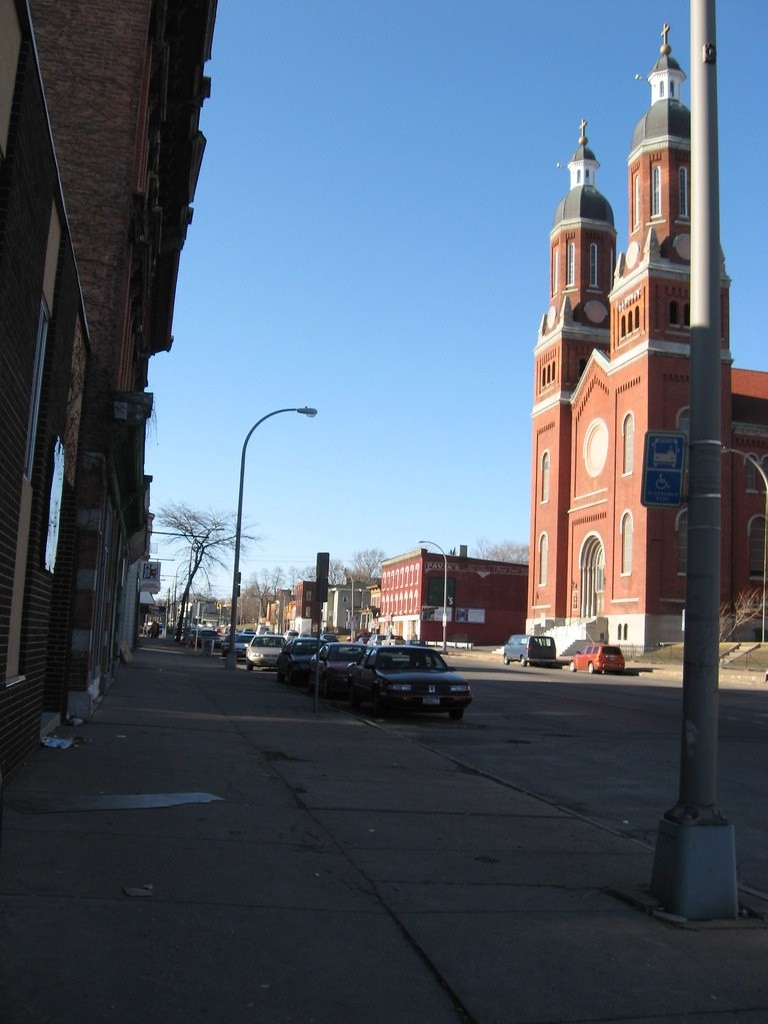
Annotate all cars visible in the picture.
[276,630,339,687]
[246,625,288,671]
[189,623,221,648]
[569,644,625,674]
[350,645,474,720]
[222,628,256,661]
[308,642,370,698]
[347,631,425,646]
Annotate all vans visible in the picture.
[503,634,557,667]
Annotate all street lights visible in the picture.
[722,447,768,643]
[333,567,354,643]
[182,528,225,648]
[417,540,448,654]
[224,407,318,669]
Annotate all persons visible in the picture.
[149,620,160,639]
